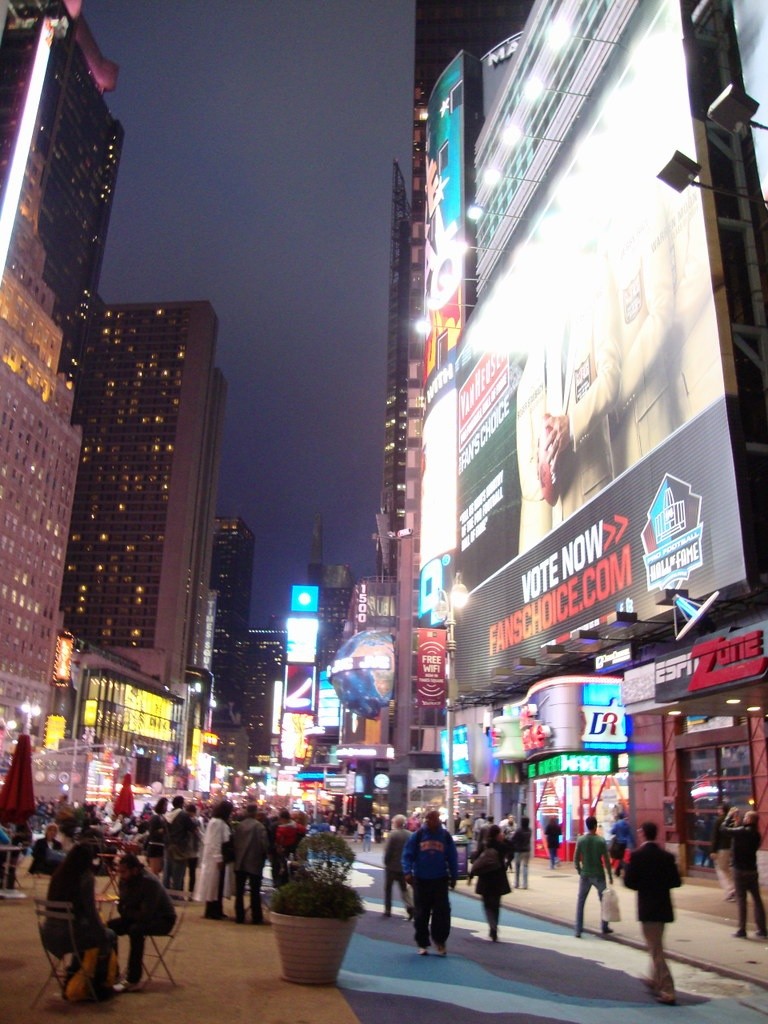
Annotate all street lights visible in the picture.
[434,570,471,834]
[20,693,41,736]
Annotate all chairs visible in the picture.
[0,839,192,1014]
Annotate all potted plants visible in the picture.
[268,880,367,988]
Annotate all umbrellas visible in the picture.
[0,734,34,889]
[115,773,136,818]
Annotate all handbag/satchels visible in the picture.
[600,884,621,921]
[467,842,506,883]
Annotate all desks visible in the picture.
[97,852,121,897]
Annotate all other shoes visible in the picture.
[603,928,615,936]
[417,947,428,955]
[436,948,446,956]
[755,929,766,936]
[733,930,746,937]
[723,888,735,902]
[490,929,498,942]
[113,978,137,994]
[408,908,415,921]
[383,912,391,917]
[204,911,265,924]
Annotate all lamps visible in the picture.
[454,657,567,695]
[657,150,768,206]
[655,589,743,607]
[571,630,662,643]
[606,611,686,626]
[415,26,619,336]
[540,644,595,660]
[708,84,768,138]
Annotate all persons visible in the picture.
[709,806,737,902]
[544,817,562,870]
[611,813,632,875]
[516,220,680,554]
[405,811,421,831]
[573,817,613,937]
[42,843,117,1000]
[137,795,314,924]
[469,826,511,939]
[453,812,516,870]
[315,810,385,852]
[623,822,683,1005]
[720,808,768,937]
[402,811,459,956]
[513,817,531,888]
[0,793,156,877]
[108,856,177,990]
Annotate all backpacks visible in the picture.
[62,927,120,1002]
[653,850,682,888]
